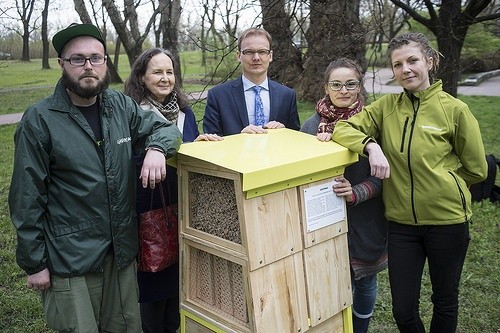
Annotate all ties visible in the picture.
[252,86,265,127]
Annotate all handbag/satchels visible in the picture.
[139,179,180,273]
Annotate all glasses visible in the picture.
[61,53,107,66]
[241,48,270,56]
[326,80,360,91]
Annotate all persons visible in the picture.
[204,28,301,137]
[9,24,182,333]
[332,33,488,333]
[125,48,223,333]
[299,58,391,333]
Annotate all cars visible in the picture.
[292,40,305,48]
[0,51,10,60]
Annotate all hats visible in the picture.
[51,22,106,55]
